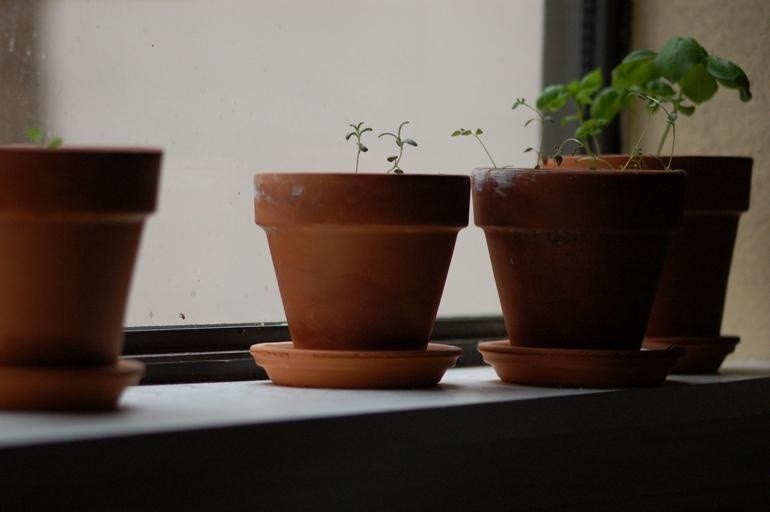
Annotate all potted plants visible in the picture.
[452,39,753,386]
[251,122,473,390]
[1,129,165,414]
